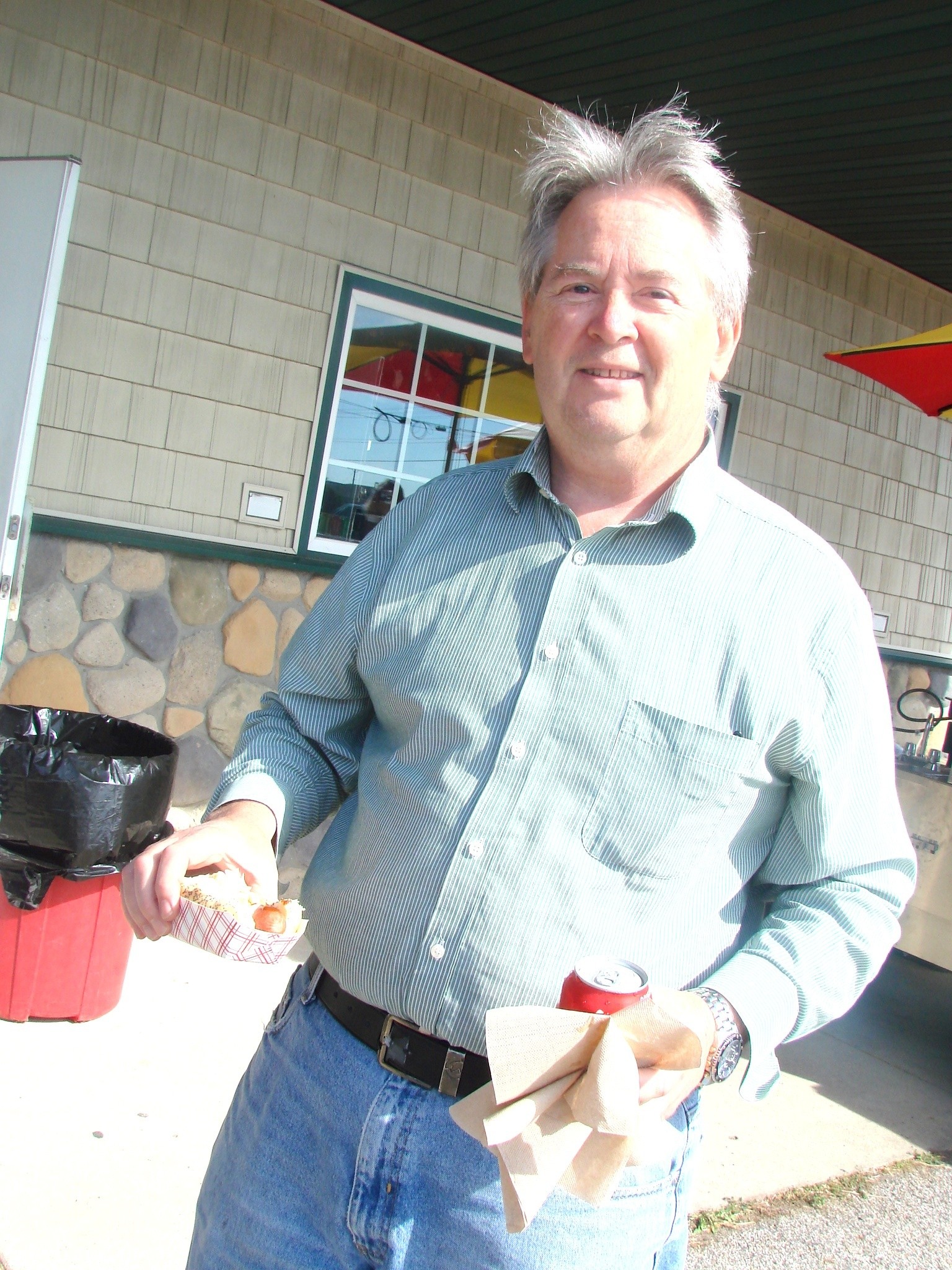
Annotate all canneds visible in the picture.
[558,957,652,1015]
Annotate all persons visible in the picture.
[116,84,918,1269]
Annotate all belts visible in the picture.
[308,951,492,1098]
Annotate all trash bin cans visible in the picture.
[0,703,179,1029]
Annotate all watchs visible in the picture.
[683,988,745,1089]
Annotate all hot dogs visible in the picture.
[170,869,308,963]
